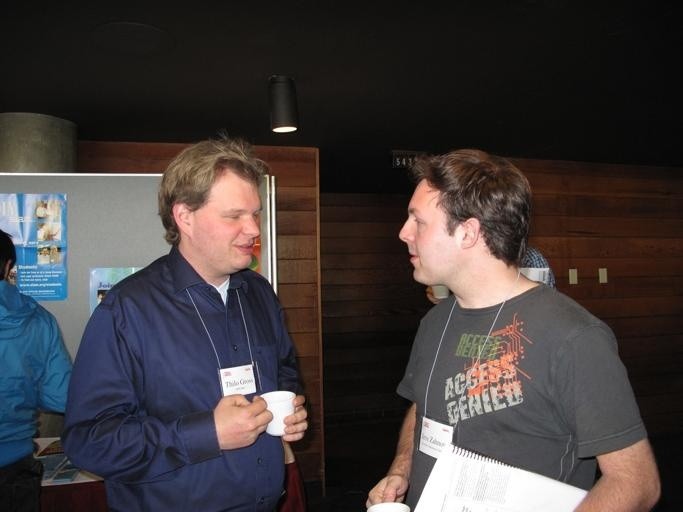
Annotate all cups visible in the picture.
[367,502,411,512]
[430,285,450,301]
[262,390,296,438]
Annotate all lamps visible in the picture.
[266,77,297,134]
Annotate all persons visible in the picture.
[0,230,72,511]
[57,138,314,510]
[358,145,662,512]
[423,245,561,306]
